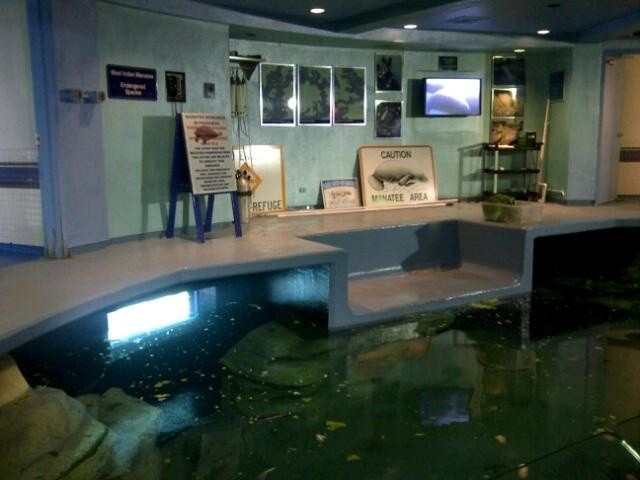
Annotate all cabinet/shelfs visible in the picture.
[483,142,542,201]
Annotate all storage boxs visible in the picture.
[480,201,544,224]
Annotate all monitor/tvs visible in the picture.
[423,78,482,117]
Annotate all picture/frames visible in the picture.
[373,99,405,141]
[319,177,361,208]
[233,142,286,218]
[258,61,368,127]
[165,69,188,104]
[355,144,438,210]
[490,54,530,143]
[373,51,404,95]
